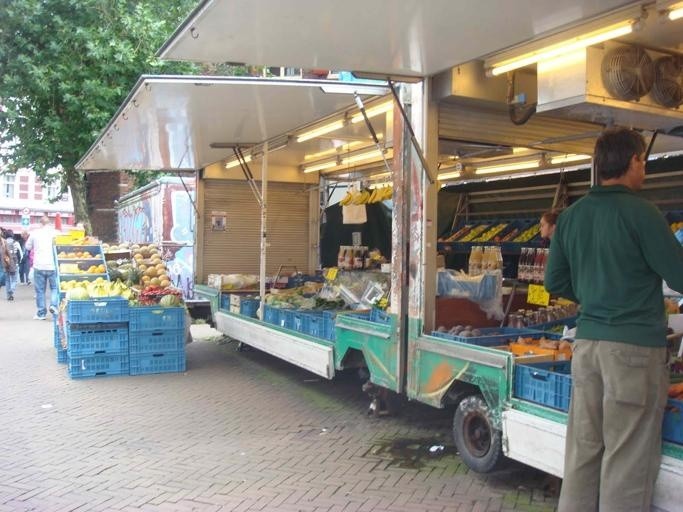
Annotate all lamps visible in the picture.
[224,136,292,171]
[650,0,683,24]
[294,110,349,146]
[478,1,657,80]
[347,91,395,126]
[298,145,592,191]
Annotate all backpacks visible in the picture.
[5,239,17,273]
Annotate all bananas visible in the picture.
[91,278,135,300]
[436,178,441,191]
[337,182,395,205]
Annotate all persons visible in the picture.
[520,247,549,283]
[0,226,33,301]
[542,124,681,512]
[23,216,61,319]
[540,211,560,242]
[118,206,149,244]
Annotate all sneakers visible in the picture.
[7,294,15,300]
[50,306,55,313]
[33,315,46,320]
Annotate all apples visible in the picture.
[130,243,172,291]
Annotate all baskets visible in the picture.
[53,291,187,379]
[240,299,257,317]
[53,242,110,297]
[430,316,547,347]
[264,304,337,342]
[660,399,683,442]
[513,359,572,413]
[221,294,230,310]
[370,308,391,324]
[527,316,578,342]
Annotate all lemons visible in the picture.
[84,263,105,275]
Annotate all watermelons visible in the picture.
[160,294,181,308]
[129,300,145,307]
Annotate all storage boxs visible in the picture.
[217,214,682,446]
[54,230,192,380]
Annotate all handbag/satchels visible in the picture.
[4,254,10,267]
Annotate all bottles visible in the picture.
[338,246,369,271]
[507,305,570,329]
[517,248,549,285]
[469,246,503,278]
